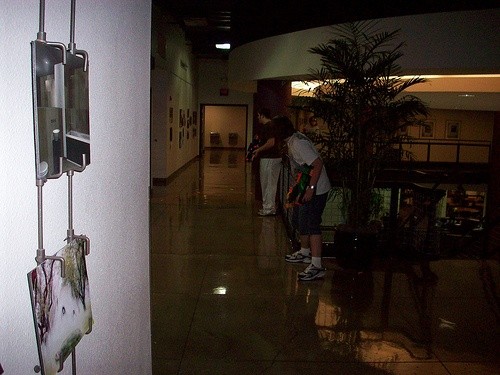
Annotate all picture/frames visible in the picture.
[419,119,435,139]
[445,120,461,140]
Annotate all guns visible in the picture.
[284,163,314,209]
[246,138,259,162]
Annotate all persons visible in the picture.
[271,115,331,280]
[253,108,282,216]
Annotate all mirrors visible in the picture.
[31,41,90,176]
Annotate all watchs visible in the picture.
[307,185,316,190]
